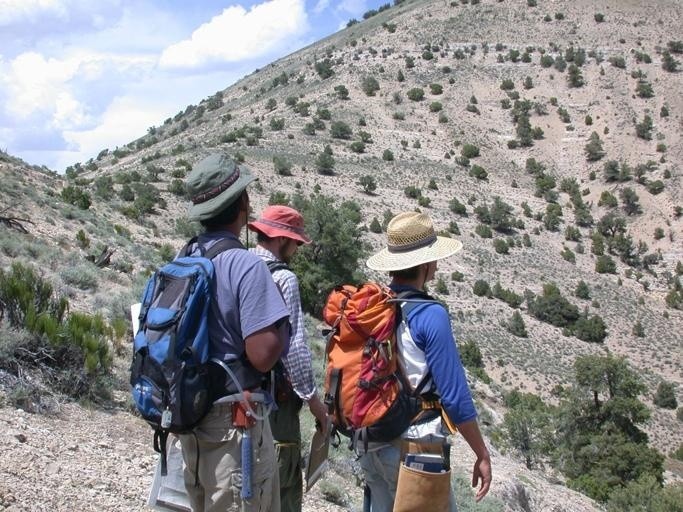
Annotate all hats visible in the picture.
[248,206,314,245]
[185,153,260,222]
[366,211,462,271]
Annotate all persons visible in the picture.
[243,204,334,511]
[144,150,294,511]
[351,210,494,512]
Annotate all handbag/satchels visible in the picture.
[393,443,451,512]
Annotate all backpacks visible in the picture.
[131,237,248,435]
[321,283,434,443]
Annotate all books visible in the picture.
[304,426,331,496]
[404,452,446,473]
